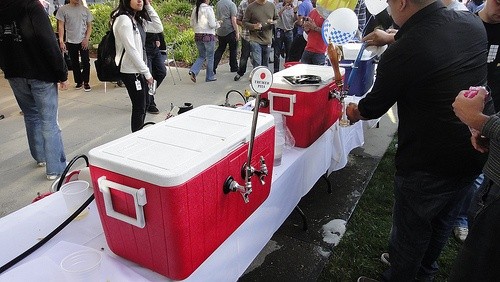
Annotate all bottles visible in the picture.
[277,29,281,38]
[239,98,257,111]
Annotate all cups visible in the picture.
[148,80,157,95]
[218,20,223,29]
[270,112,285,167]
[257,22,263,32]
[304,21,311,31]
[337,102,351,127]
[60,250,103,282]
[60,181,89,221]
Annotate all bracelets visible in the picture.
[314,27,320,31]
[294,11,297,14]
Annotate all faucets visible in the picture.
[240,155,269,186]
[229,166,252,204]
[329,89,349,102]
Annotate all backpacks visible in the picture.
[94,11,136,82]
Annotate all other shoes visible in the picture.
[46,162,73,180]
[84,83,92,91]
[231,67,239,72]
[37,161,46,166]
[454,227,468,244]
[381,253,434,282]
[206,76,217,82]
[148,104,159,114]
[75,83,82,89]
[234,75,241,81]
[189,72,197,83]
[357,276,377,282]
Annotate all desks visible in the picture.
[0,64,386,282]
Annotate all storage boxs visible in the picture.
[87,105,275,281]
[326,42,388,97]
[257,63,345,148]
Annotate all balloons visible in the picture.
[315,0,358,45]
[364,0,389,16]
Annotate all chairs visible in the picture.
[158,40,182,85]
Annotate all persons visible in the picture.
[189,0,224,83]
[0,0,72,180]
[111,0,167,133]
[213,0,240,74]
[234,0,343,81]
[39,0,94,91]
[346,0,500,282]
